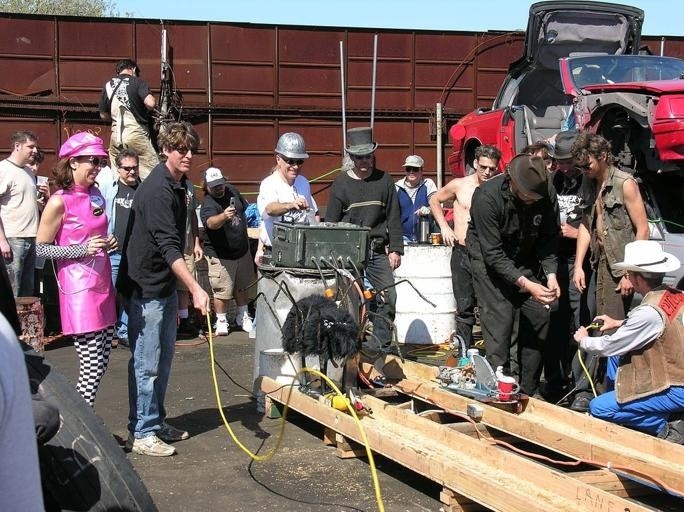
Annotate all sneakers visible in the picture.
[179,318,195,331]
[571,391,593,412]
[118,338,130,351]
[157,422,189,441]
[236,312,253,333]
[126,432,176,456]
[213,319,229,336]
[657,416,684,445]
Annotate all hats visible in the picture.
[59,131,108,159]
[508,153,552,199]
[345,127,378,156]
[403,155,424,167]
[548,130,579,159]
[205,166,224,188]
[614,240,680,273]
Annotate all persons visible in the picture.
[394,153,435,246]
[97,59,158,183]
[325,126,402,365]
[427,133,683,444]
[200,167,259,334]
[0,119,212,456]
[253,133,319,266]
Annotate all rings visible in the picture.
[89,243,95,248]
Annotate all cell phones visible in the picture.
[229,197,235,208]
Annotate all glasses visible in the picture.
[406,167,419,172]
[478,164,496,171]
[177,147,196,154]
[574,162,593,172]
[356,155,371,160]
[88,156,107,167]
[281,157,304,165]
[121,166,138,171]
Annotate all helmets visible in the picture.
[275,132,309,159]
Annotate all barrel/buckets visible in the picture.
[384,242,458,346]
[252,262,355,400]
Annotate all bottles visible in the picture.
[416,212,430,245]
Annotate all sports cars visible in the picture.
[448,2,683,194]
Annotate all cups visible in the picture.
[426,233,442,248]
[497,376,515,401]
